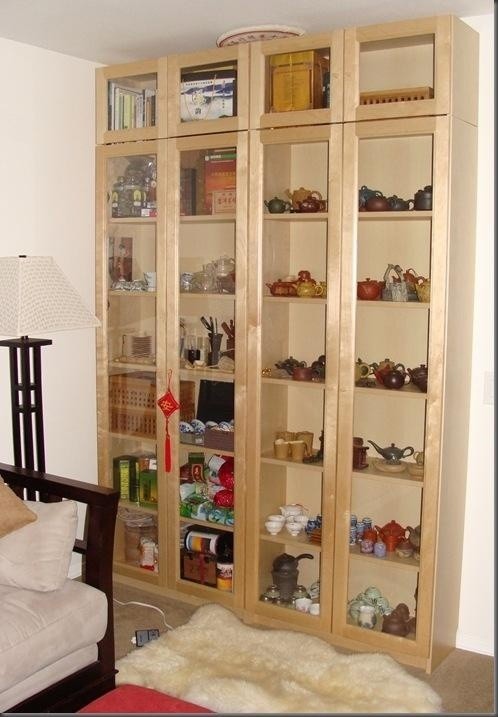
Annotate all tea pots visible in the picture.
[375,519,420,545]
[274,354,428,394]
[367,439,415,465]
[382,612,411,638]
[264,184,433,214]
[266,264,431,303]
[279,504,309,516]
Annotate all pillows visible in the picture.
[0,498,80,593]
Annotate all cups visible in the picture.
[273,430,315,461]
[358,605,377,630]
[295,598,320,616]
[413,451,424,466]
[351,514,386,555]
[188,349,200,365]
[143,271,155,292]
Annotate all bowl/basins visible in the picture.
[264,515,308,537]
[179,418,205,434]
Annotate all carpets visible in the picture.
[115,599,445,714]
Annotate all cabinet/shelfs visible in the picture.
[92,10,482,677]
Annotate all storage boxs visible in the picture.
[177,547,230,587]
[269,50,329,112]
[108,370,197,440]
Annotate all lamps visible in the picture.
[0,252,105,472]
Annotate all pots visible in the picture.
[273,550,313,573]
